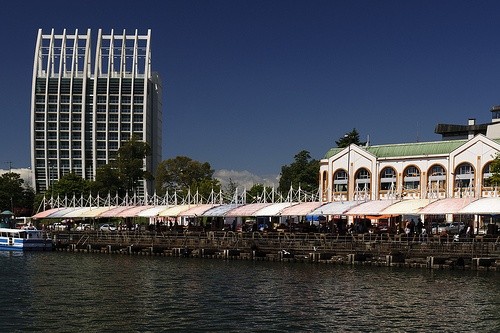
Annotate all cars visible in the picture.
[478,224,488,235]
[46,222,139,232]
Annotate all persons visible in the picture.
[152,217,475,239]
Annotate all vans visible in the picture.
[420,222,465,236]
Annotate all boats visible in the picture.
[0,225,54,251]
